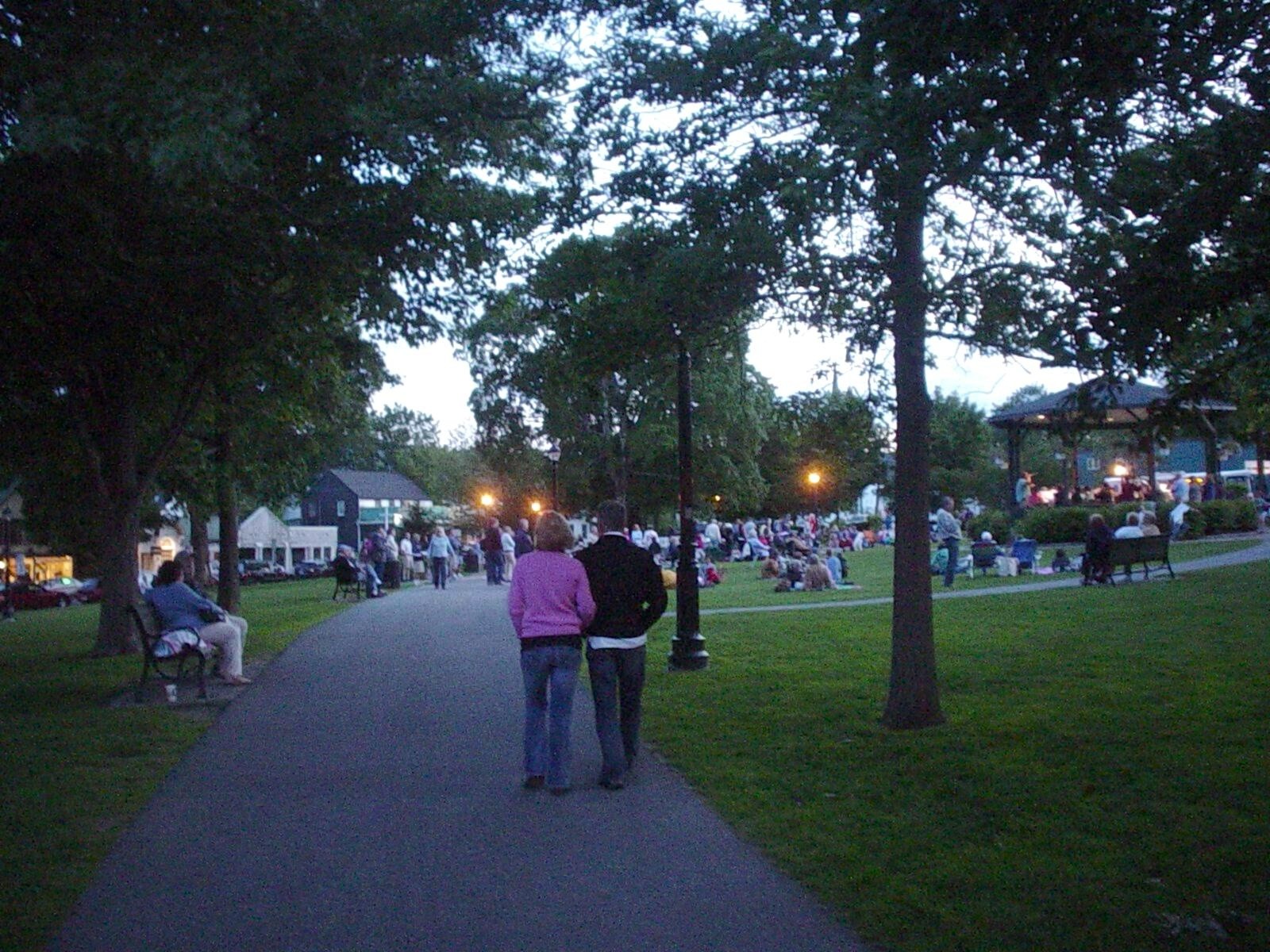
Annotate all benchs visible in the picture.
[128,596,229,701]
[329,562,372,600]
[1089,533,1176,584]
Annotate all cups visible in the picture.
[165,682,178,702]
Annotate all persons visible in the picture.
[574,501,895,593]
[927,522,1005,578]
[508,511,597,795]
[172,548,249,681]
[937,492,983,588]
[330,515,535,599]
[144,561,253,688]
[574,500,668,791]
[1011,470,1270,582]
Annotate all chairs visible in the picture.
[968,541,998,580]
[1005,539,1042,572]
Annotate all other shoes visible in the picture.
[225,676,252,685]
[217,672,230,680]
[525,775,544,788]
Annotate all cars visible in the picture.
[1097,469,1270,525]
[0,573,102,610]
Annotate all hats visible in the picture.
[174,550,196,566]
